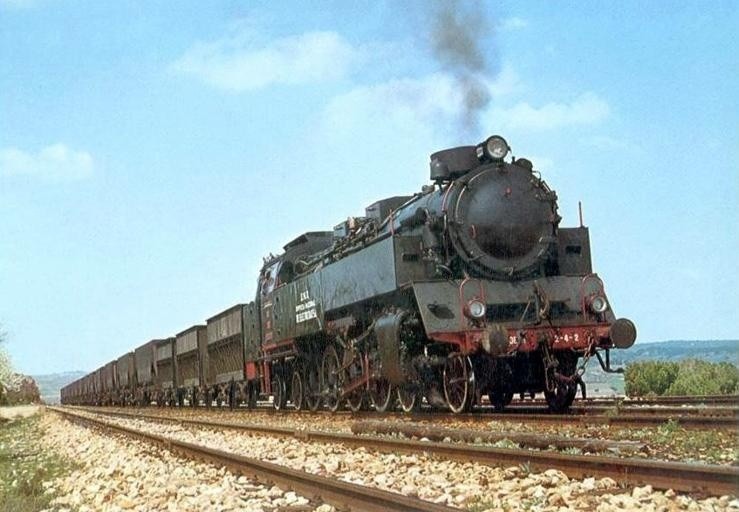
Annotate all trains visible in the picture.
[61,136,635,416]
